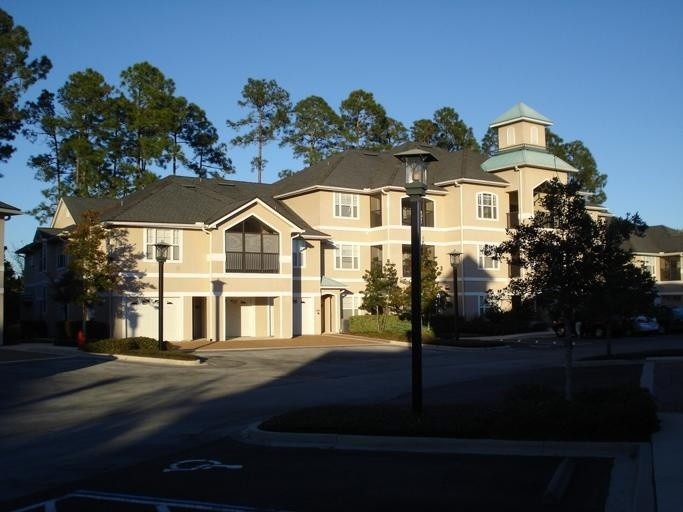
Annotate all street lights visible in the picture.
[445,249,464,341]
[392,147,439,421]
[150,239,172,351]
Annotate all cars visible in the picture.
[550,310,630,340]
[629,314,658,334]
[656,303,682,329]
